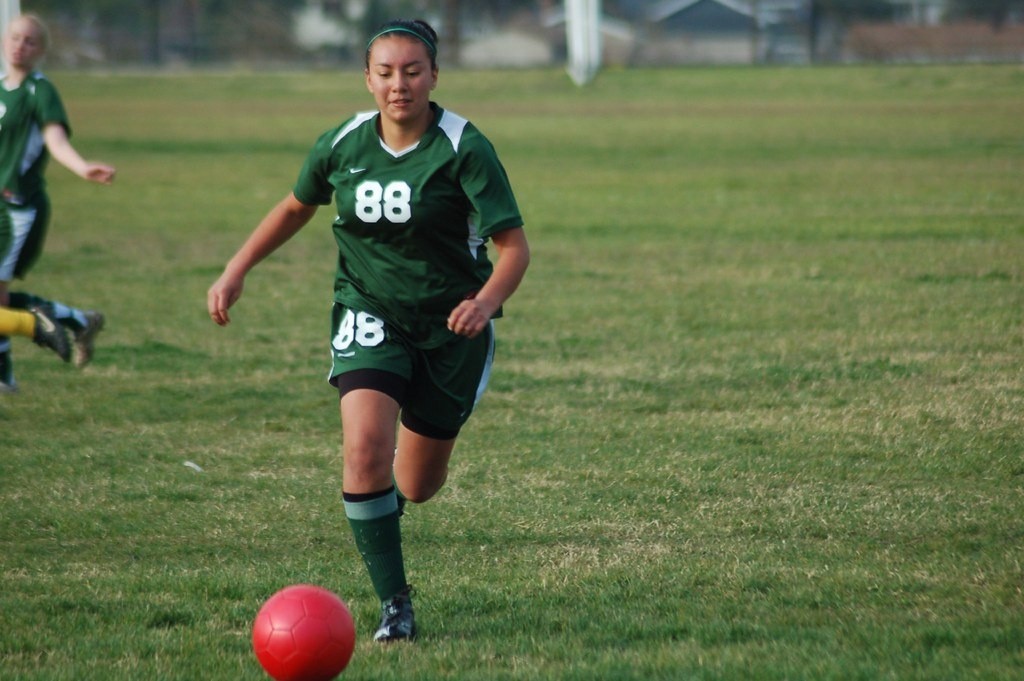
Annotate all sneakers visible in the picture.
[75,311,106,365]
[373,585,416,640]
[29,303,72,363]
[0,371,17,391]
[396,493,405,515]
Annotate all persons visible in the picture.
[205,17,532,642]
[0,14,116,394]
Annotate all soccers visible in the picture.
[252,582,357,681]
